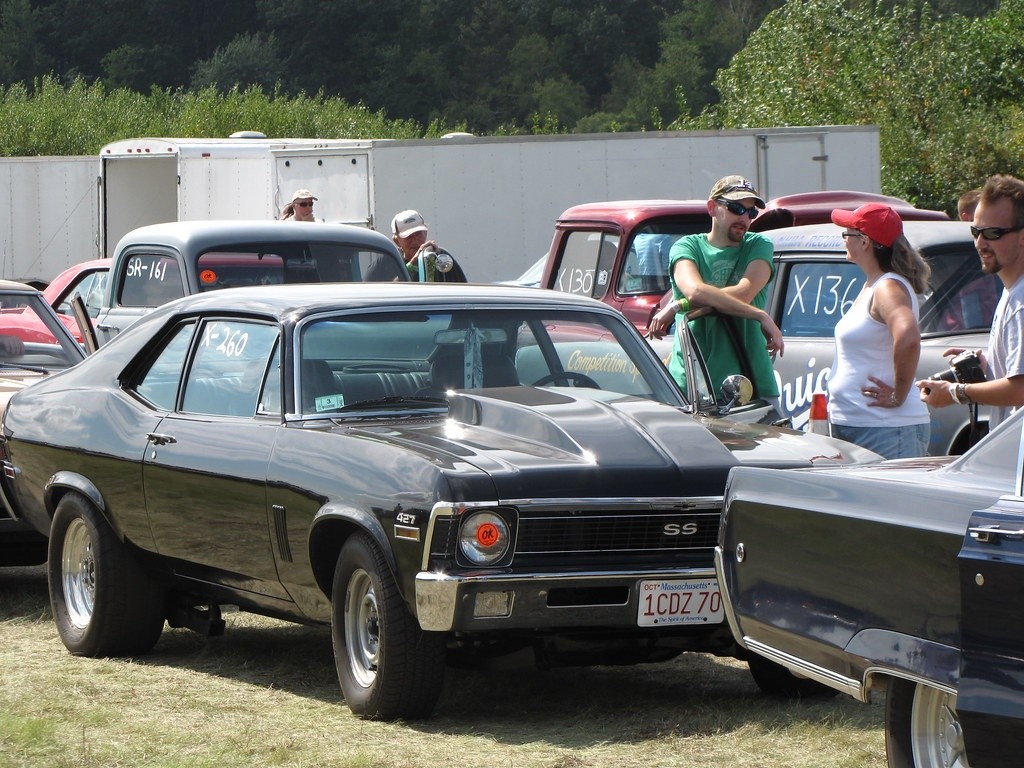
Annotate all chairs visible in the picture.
[241,359,336,414]
[429,354,520,392]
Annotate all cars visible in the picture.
[714,403,1024,768]
[1,222,887,723]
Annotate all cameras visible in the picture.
[924,351,987,395]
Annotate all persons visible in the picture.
[362,210,468,282]
[915,174,1024,433]
[633,234,674,291]
[279,189,323,223]
[958,190,981,222]
[826,203,931,460]
[649,175,784,419]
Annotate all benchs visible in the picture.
[184,370,430,417]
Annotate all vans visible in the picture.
[483,191,1015,454]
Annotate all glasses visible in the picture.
[970,225,1024,240]
[842,231,866,239]
[295,202,314,207]
[716,198,758,219]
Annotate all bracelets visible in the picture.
[889,392,895,402]
[406,262,418,272]
[949,383,962,404]
[958,384,970,404]
[672,301,680,312]
[680,298,689,311]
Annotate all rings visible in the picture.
[875,393,877,397]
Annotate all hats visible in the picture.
[391,210,428,240]
[292,189,318,201]
[831,202,903,248]
[709,175,765,209]
